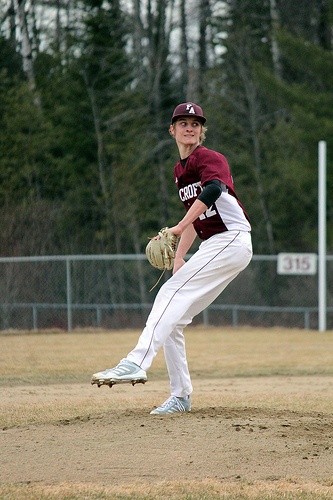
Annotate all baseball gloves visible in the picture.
[146,227,177,292]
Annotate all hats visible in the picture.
[172,102,207,126]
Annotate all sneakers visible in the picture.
[91,359,148,388]
[150,397,191,416]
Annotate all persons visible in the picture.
[90,101,253,416]
